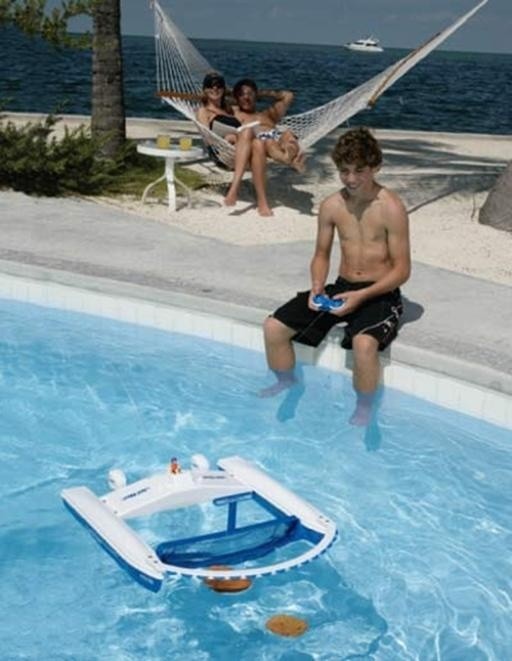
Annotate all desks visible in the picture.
[136,140,203,214]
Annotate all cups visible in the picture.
[179,136,193,152]
[156,133,171,151]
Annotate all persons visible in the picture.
[258,126,413,426]
[197,73,273,217]
[221,80,315,176]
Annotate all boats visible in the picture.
[344,34,384,52]
